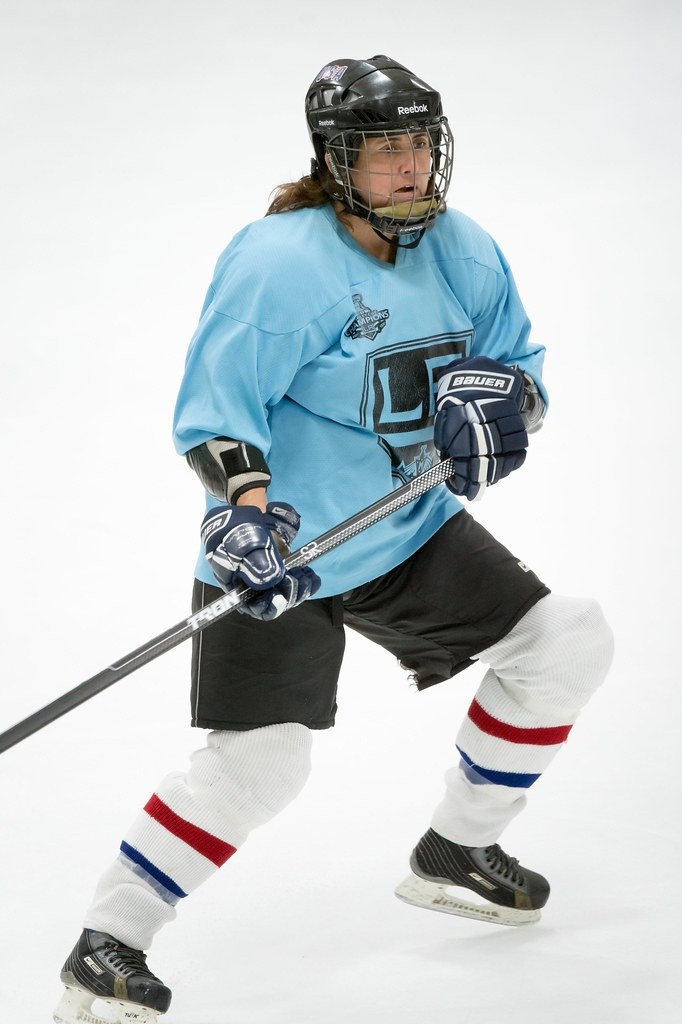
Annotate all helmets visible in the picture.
[302,54,456,250]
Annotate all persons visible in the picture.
[61,56,618,1016]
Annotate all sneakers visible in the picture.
[56,927,170,1024]
[397,828,549,926]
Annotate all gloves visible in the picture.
[199,501,321,622]
[432,356,529,505]
